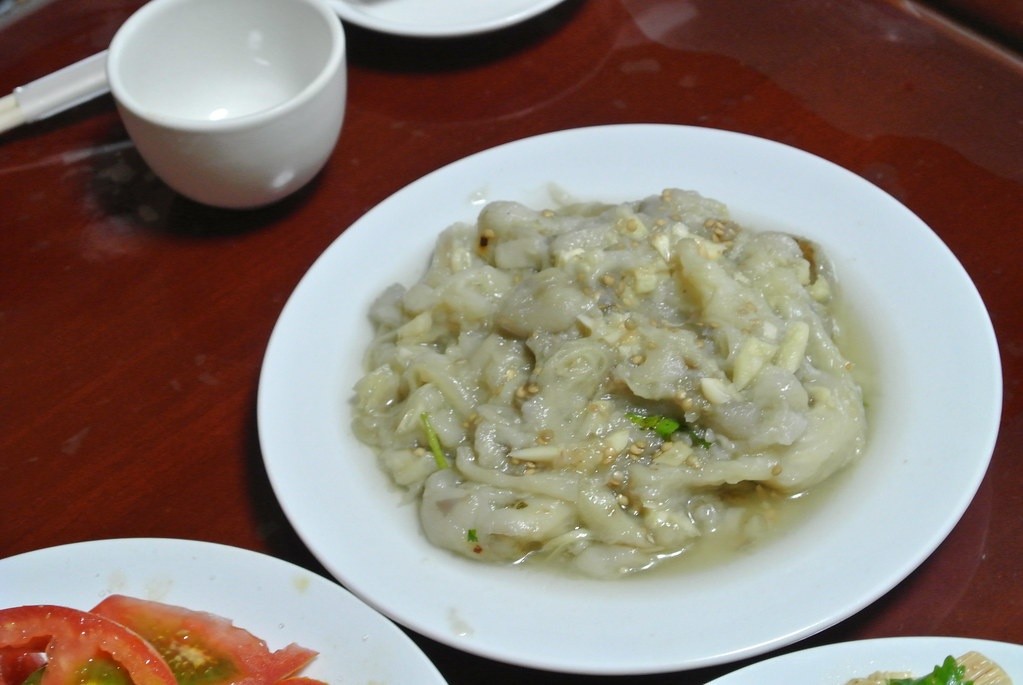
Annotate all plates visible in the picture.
[701,634,1023,685]
[257,124,1005,677]
[0,537,447,685]
[329,0,563,40]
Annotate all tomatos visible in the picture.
[0,594,331,685]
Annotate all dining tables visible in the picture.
[0,0,1023,685]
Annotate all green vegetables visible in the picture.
[421,409,711,469]
[887,654,974,685]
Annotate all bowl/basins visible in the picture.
[106,0,349,212]
